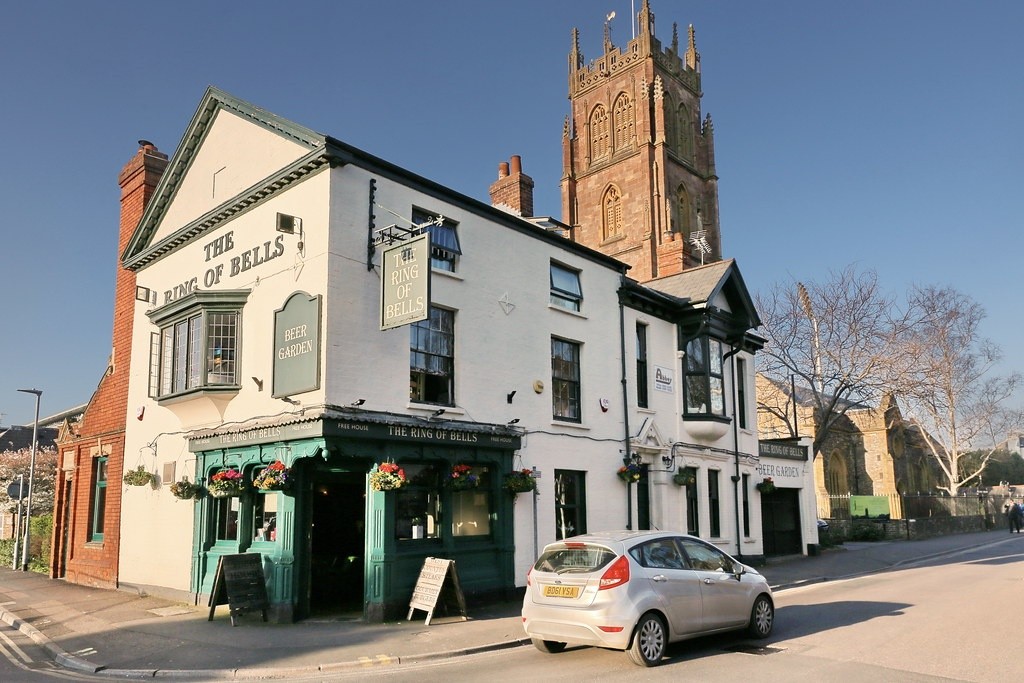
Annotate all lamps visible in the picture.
[432,408,444,418]
[253,376,262,390]
[633,454,643,464]
[662,456,672,468]
[282,396,299,406]
[508,418,519,426]
[351,399,365,408]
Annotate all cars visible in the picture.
[522,530,774,667]
[817,518,829,532]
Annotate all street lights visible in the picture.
[17,388,43,572]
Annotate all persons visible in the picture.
[1018,500,1024,530]
[1009,498,1020,533]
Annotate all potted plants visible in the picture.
[756,478,777,496]
[673,473,695,486]
[124,465,156,490]
[170,475,199,501]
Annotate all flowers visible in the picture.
[206,469,245,498]
[618,463,640,482]
[252,461,289,490]
[452,465,473,478]
[371,464,409,491]
[506,468,541,495]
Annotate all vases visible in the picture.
[455,475,471,485]
[258,487,285,494]
[511,481,533,492]
[625,474,637,483]
[213,489,239,497]
